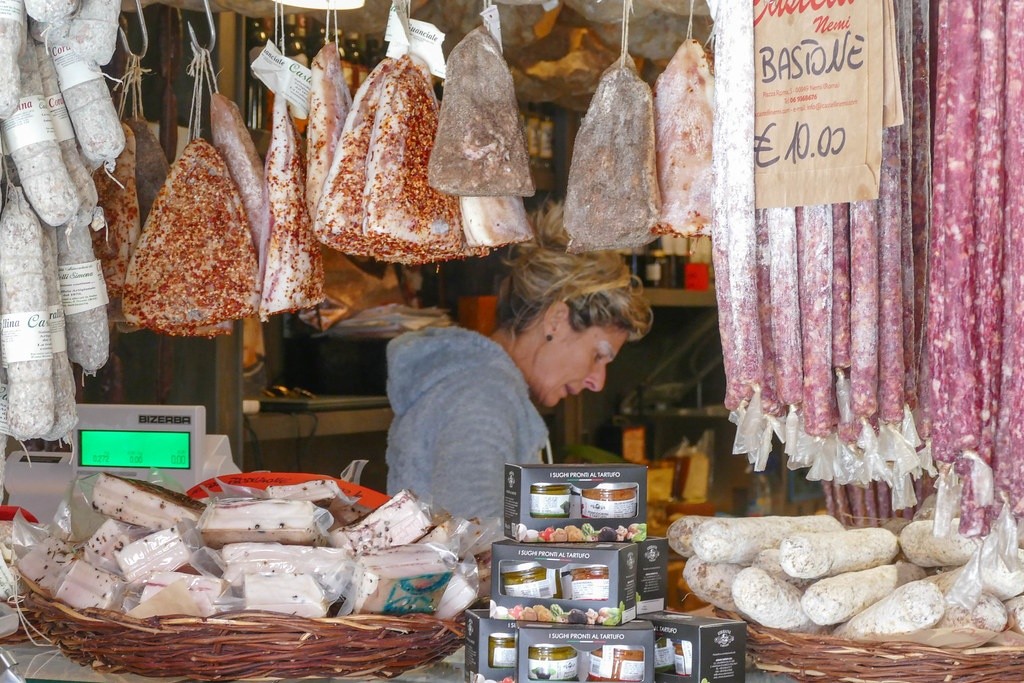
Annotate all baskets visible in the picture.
[15,496,489,683]
[712,606,1024,683]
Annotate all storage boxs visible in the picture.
[638,610,747,683]
[634,537,667,615]
[464,608,656,683]
[488,537,638,627]
[503,463,649,545]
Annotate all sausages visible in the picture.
[716,0,1024,522]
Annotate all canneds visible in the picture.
[568,564,608,600]
[488,633,516,667]
[646,250,667,286]
[671,639,692,677]
[588,645,643,682]
[501,561,553,598]
[654,636,675,674]
[529,644,578,681]
[582,483,636,518]
[530,483,571,519]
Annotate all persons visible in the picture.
[382,201,653,534]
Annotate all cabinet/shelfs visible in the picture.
[203,9,829,516]
